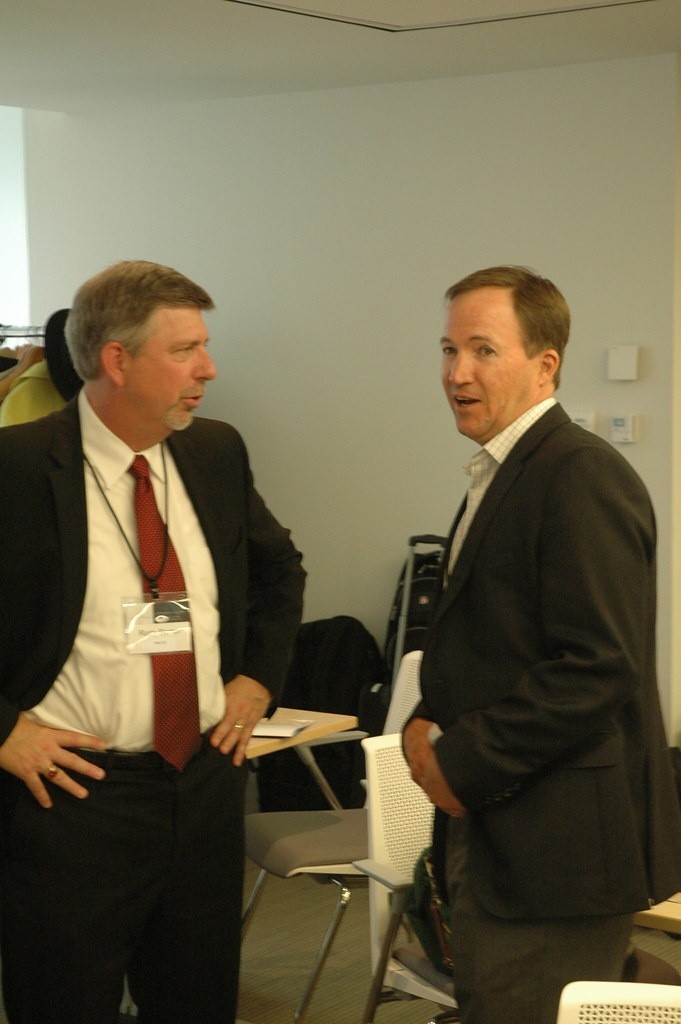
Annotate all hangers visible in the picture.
[0,323,46,402]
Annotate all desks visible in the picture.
[242,707,357,761]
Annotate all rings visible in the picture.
[232,724,245,729]
[47,764,58,779]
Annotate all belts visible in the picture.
[64,728,217,771]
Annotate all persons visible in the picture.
[0,259,309,1024]
[0,308,43,406]
[399,264,681,1024]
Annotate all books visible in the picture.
[250,718,312,738]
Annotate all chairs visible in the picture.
[361,724,458,1024]
[241,650,423,1024]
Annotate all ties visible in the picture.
[126,452,201,770]
